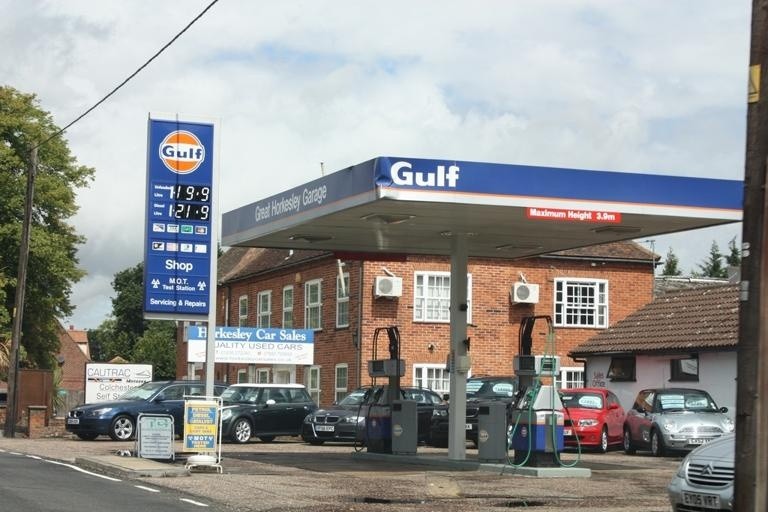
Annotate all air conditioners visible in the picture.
[373,277,402,298]
[511,283,539,304]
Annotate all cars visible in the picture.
[213,383,321,445]
[302,386,445,446]
[559,387,627,452]
[668,431,736,511]
[428,380,548,447]
[623,386,736,456]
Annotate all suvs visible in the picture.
[67,379,234,442]
